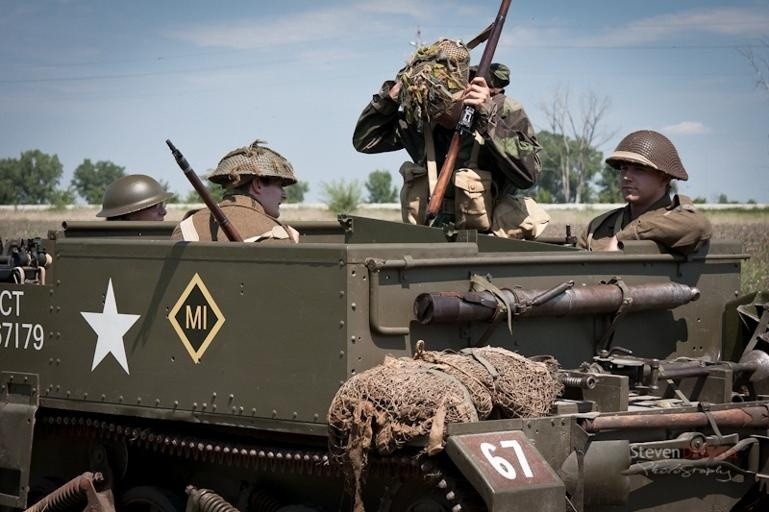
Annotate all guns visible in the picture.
[425,0,510,219]
[562,224,577,247]
[165,139,244,242]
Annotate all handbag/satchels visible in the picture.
[453,168,494,232]
[399,160,429,225]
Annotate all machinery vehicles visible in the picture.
[1,219,769,512]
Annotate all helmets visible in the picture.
[208,140,297,186]
[606,131,688,181]
[398,39,470,117]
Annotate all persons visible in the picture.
[577,130,714,251]
[352,37,543,241]
[169,145,300,242]
